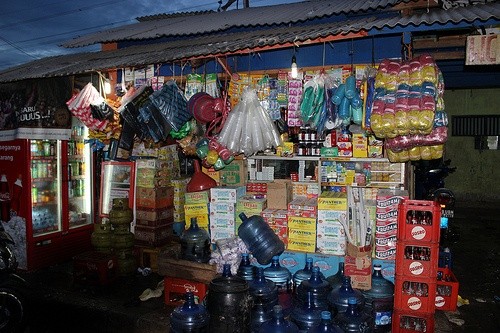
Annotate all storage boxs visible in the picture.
[72,251,119,288]
[134,132,459,333]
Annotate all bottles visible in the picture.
[289,292,323,333]
[308,310,344,333]
[230,253,258,287]
[335,297,375,333]
[298,267,330,306]
[262,256,292,317]
[30,140,89,235]
[248,266,280,329]
[327,276,365,319]
[255,304,299,332]
[237,212,284,265]
[179,217,211,265]
[401,188,456,332]
[169,291,207,333]
[295,259,325,287]
[206,263,252,333]
[324,262,345,293]
[360,263,394,333]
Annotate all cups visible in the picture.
[215,102,281,156]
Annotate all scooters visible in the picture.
[0,219,37,333]
[416,159,461,245]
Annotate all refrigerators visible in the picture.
[0,127,96,274]
